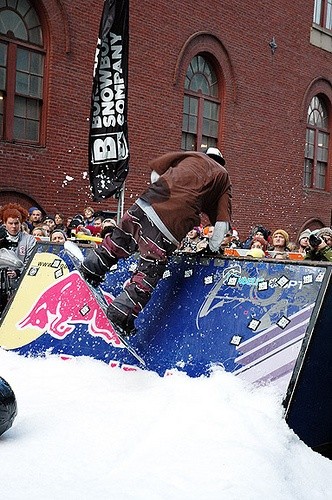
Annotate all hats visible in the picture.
[298,228,311,240]
[205,146,226,166]
[272,229,289,243]
[316,227,331,239]
[256,226,268,240]
[28,207,39,214]
[40,215,55,225]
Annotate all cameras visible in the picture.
[309,234,328,246]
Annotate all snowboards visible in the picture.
[63,240,148,368]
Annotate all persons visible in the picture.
[79,147,233,337]
[0,202,117,317]
[175,224,332,262]
